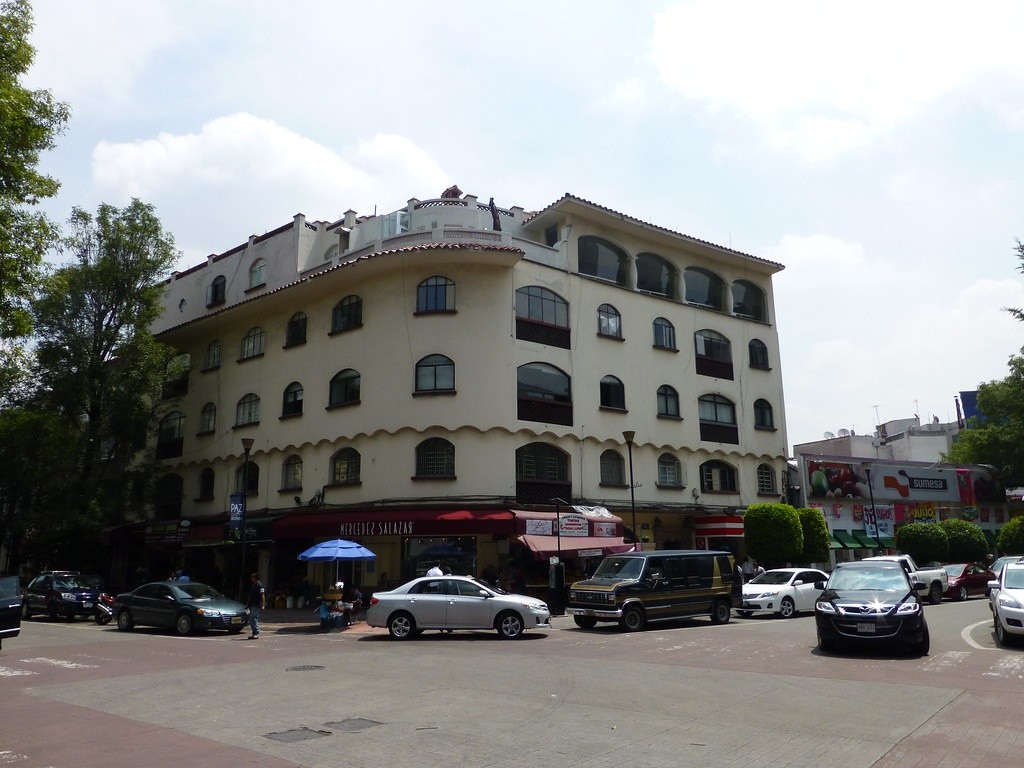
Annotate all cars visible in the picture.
[366,576,551,640]
[0,575,22,650]
[111,581,251,637]
[987,562,1024,647]
[734,568,831,619]
[988,555,1024,580]
[20,569,101,623]
[813,560,930,657]
[940,563,997,602]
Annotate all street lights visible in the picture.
[621,430,637,551]
[239,437,256,603]
[861,460,881,551]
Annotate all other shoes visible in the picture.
[248,633,259,639]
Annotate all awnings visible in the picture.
[833,530,862,550]
[984,531,998,547]
[852,530,897,549]
[828,534,843,550]
[509,508,642,561]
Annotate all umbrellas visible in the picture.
[297,538,377,582]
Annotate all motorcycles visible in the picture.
[94,591,115,625]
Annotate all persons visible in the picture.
[347,584,363,625]
[776,574,785,584]
[742,554,754,582]
[496,567,516,593]
[178,570,190,595]
[443,567,453,594]
[736,565,744,585]
[137,566,156,588]
[291,565,308,598]
[580,559,596,580]
[167,571,177,582]
[426,560,443,592]
[479,563,496,587]
[752,561,765,577]
[272,509,517,536]
[538,561,561,616]
[209,566,224,592]
[245,572,265,639]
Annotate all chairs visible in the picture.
[351,600,367,626]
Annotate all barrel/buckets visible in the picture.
[286,596,294,609]
[297,599,303,608]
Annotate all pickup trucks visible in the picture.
[862,554,949,605]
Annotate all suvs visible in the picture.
[563,549,744,633]
